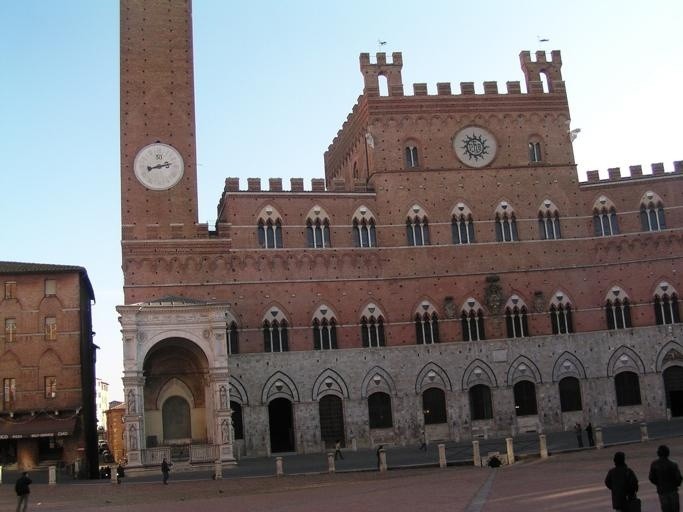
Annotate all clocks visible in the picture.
[134,143,184,191]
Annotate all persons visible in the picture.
[333,440,344,460]
[573,421,583,447]
[105,464,111,479]
[585,421,595,446]
[103,466,106,479]
[99,466,104,479]
[418,431,427,451]
[648,444,683,511]
[376,445,384,468]
[14,471,32,511]
[603,451,642,512]
[116,463,124,478]
[160,457,173,485]
